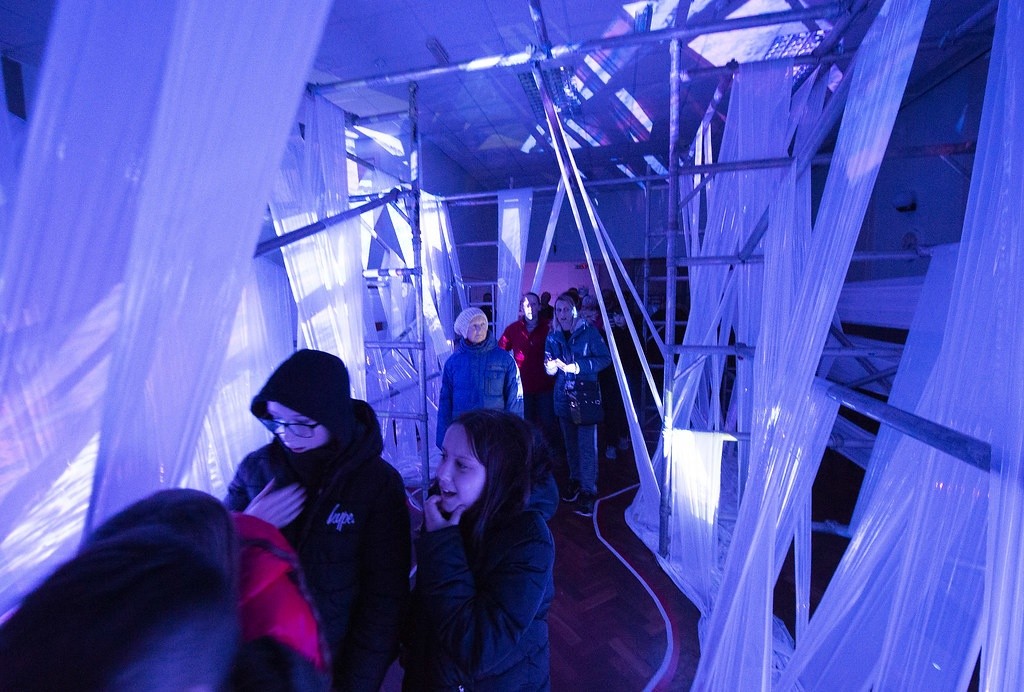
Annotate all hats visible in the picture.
[0,488,239,692]
[454,307,488,339]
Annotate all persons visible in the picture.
[220,348,413,692]
[0,487,335,692]
[481,286,686,516]
[435,307,524,451]
[398,407,556,690]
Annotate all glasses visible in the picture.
[259,417,320,437]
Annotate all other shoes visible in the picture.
[563,478,580,501]
[605,446,616,458]
[573,490,597,516]
[618,432,629,450]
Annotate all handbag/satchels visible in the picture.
[564,379,604,426]
